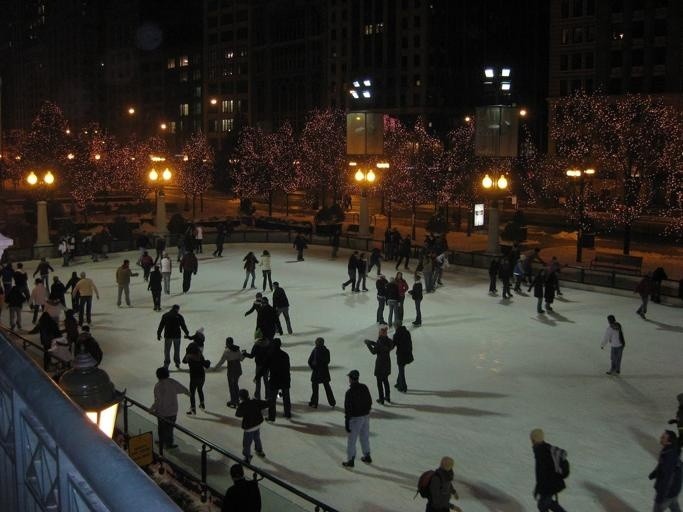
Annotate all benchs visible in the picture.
[589,252,643,277]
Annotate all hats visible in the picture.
[520,254,527,261]
[379,327,388,337]
[441,456,454,470]
[228,463,245,475]
[346,370,359,377]
[531,429,544,443]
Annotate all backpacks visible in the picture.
[418,470,434,498]
[550,445,570,479]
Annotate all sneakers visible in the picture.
[342,458,356,468]
[412,320,422,328]
[307,402,318,408]
[502,296,510,300]
[537,310,545,313]
[361,455,373,464]
[155,442,178,448]
[264,417,275,424]
[606,371,621,376]
[282,414,291,418]
[509,294,513,297]
[352,288,355,292]
[636,309,647,320]
[162,364,170,369]
[355,288,360,292]
[329,403,335,408]
[242,456,253,461]
[254,393,262,401]
[225,401,240,409]
[393,382,410,393]
[186,410,196,416]
[384,396,391,403]
[342,283,346,291]
[174,362,181,369]
[257,453,266,457]
[375,398,385,404]
[362,287,369,292]
[376,320,389,325]
[198,403,205,411]
[546,306,553,312]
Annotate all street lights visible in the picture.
[57,325,122,443]
[565,166,596,263]
[473,68,520,268]
[145,167,172,247]
[27,171,54,260]
[345,80,384,247]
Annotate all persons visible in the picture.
[633,271,653,319]
[668,393,683,436]
[307,337,336,409]
[222,464,262,511]
[150,368,191,449]
[243,250,273,291]
[331,218,449,327]
[342,370,372,467]
[425,456,463,512]
[648,429,683,511]
[600,315,626,374]
[530,428,566,511]
[214,281,293,464]
[650,266,667,303]
[363,327,394,403]
[0,222,227,372]
[182,342,211,415]
[489,239,565,315]
[293,229,310,262]
[393,320,414,392]
